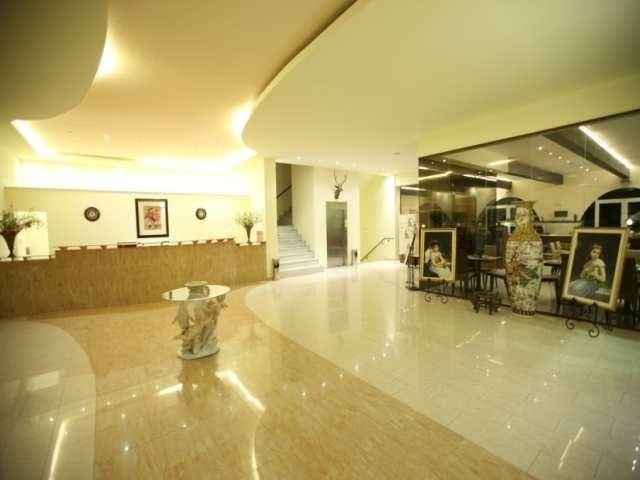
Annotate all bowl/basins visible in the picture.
[185,281,207,290]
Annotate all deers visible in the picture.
[332,170,348,200]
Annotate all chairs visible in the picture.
[456,244,507,297]
[604,256,639,325]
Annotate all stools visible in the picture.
[542,276,560,306]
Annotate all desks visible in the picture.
[544,260,562,303]
[160,283,231,360]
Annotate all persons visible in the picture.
[569,243,607,298]
[426,240,452,279]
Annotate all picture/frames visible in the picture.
[560,227,630,312]
[135,198,169,238]
[418,226,458,283]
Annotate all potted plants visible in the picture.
[0,201,45,257]
[235,210,265,243]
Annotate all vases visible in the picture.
[504,200,544,319]
